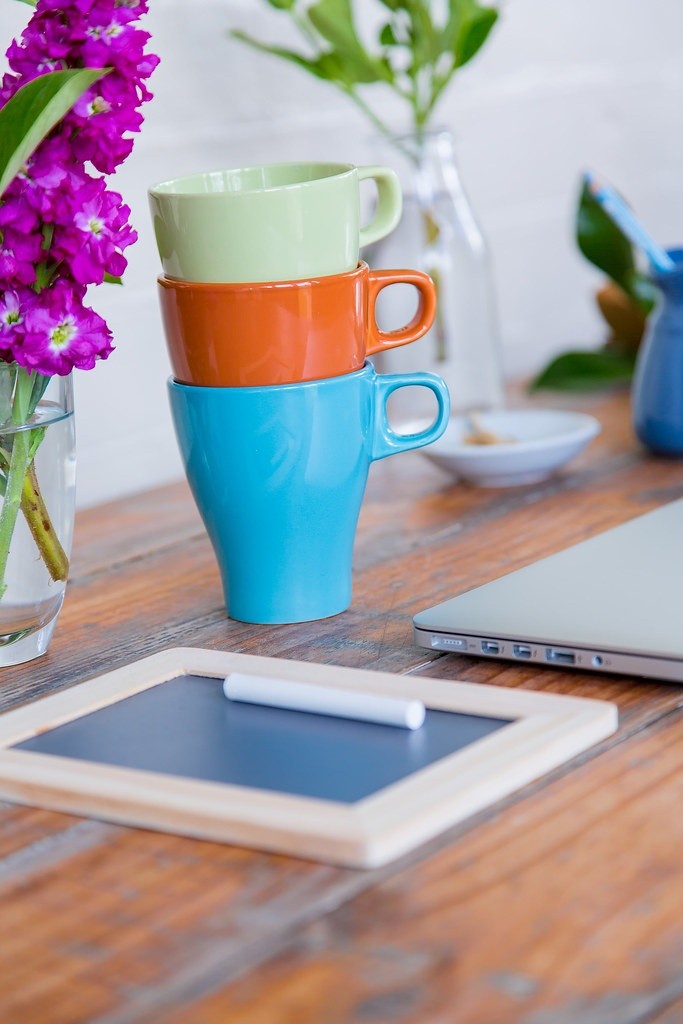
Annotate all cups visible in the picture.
[157,260,436,387]
[168,361,451,623]
[146,160,403,284]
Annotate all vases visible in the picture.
[363,122,502,498]
[0,357,74,670]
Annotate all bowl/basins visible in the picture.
[392,409,599,487]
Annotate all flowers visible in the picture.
[0,0,160,585]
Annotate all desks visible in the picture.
[0,379,683,1024]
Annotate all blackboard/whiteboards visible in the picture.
[0,645,618,877]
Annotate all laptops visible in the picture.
[413,496,683,680]
